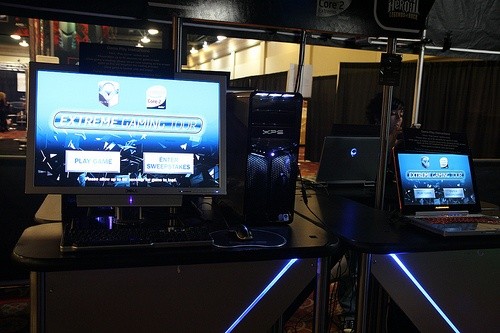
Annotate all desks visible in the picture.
[12,193,500,333]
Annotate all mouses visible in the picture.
[229,223,255,241]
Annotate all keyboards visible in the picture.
[58,218,214,251]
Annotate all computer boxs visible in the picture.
[221,86,304,224]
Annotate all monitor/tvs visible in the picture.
[24,61,227,206]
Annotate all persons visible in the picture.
[0,92,27,132]
[337,95,409,333]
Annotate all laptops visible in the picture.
[394,152,500,237]
[297,135,382,190]
